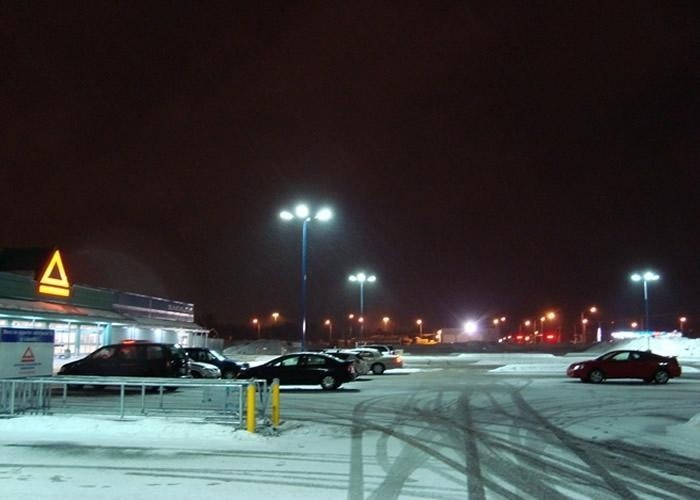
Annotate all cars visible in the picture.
[566,347,685,384]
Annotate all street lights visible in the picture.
[679,317,686,334]
[279,201,334,353]
[630,270,660,334]
[252,310,425,346]
[347,272,376,341]
[492,306,599,344]
